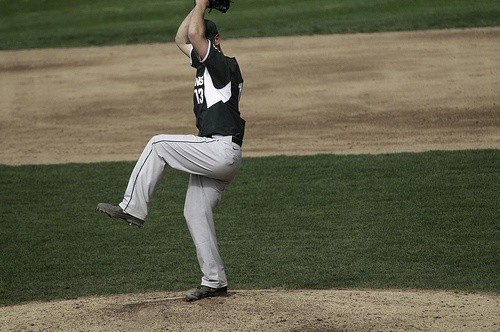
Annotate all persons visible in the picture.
[96,0,247,301]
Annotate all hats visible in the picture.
[186,19,217,44]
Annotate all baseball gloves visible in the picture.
[193,0,231,14]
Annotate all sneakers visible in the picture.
[185,286,227,302]
[96,203,144,227]
